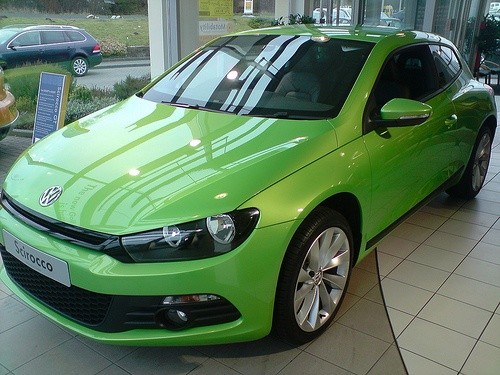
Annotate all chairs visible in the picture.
[482,58,500,85]
[273,60,322,103]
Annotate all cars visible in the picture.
[1,65,21,141]
[329,6,393,27]
[312,7,335,24]
[0,21,497,350]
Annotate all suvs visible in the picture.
[0,23,103,77]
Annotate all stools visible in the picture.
[476,69,491,86]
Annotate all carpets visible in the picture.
[487,83,500,95]
